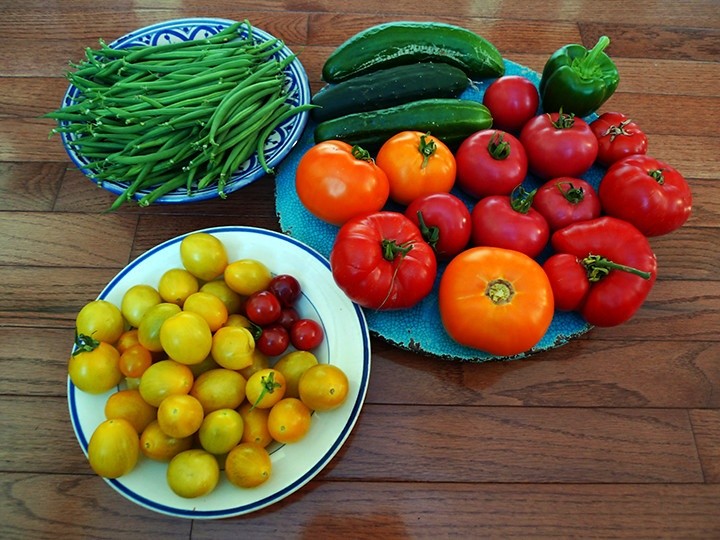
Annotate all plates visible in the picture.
[59,18,310,202]
[67,226,372,517]
[274,59,597,362]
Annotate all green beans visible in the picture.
[38,19,323,216]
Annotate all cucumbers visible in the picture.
[309,62,470,122]
[321,22,506,85]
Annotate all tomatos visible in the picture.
[296,74,692,358]
[68,233,350,498]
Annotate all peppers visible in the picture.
[538,35,620,118]
